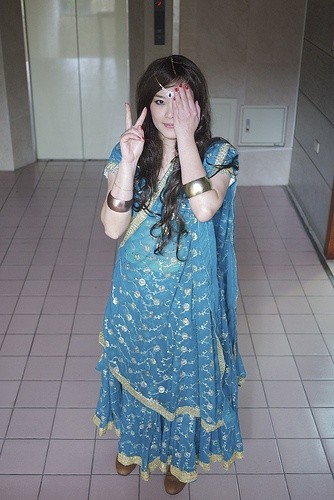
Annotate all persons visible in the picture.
[92,55,246,494]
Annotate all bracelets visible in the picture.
[114,181,134,191]
[182,176,212,199]
[107,189,134,212]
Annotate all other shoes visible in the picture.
[164,465,187,495]
[116,453,137,476]
